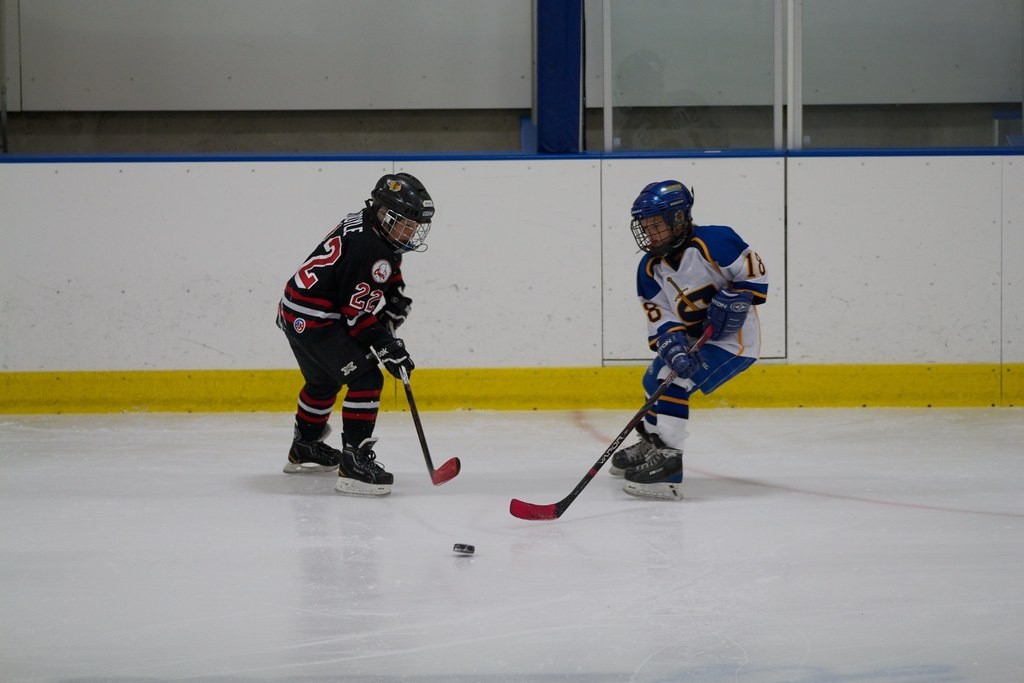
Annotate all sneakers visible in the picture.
[333,434,395,496]
[282,420,343,474]
[623,436,685,501]
[609,421,667,476]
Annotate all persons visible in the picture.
[609,179,768,501]
[275,172,435,495]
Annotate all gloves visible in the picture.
[658,334,706,382]
[369,326,414,382]
[698,286,751,341]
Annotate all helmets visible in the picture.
[629,180,693,228]
[365,171,435,227]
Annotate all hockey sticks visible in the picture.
[387,319,461,487]
[509,325,716,521]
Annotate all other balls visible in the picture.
[452,542,477,554]
